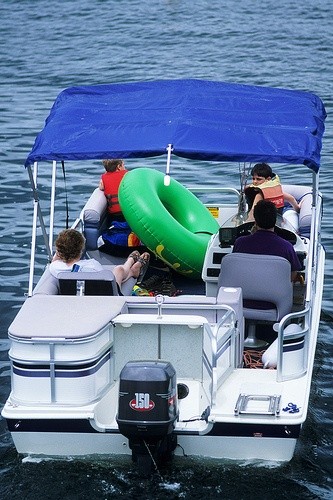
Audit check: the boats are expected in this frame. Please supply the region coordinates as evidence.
[1,78,328,481]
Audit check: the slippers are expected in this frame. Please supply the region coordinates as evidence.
[127,250,150,276]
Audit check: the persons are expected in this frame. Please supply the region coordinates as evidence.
[50,229,150,296]
[244,162,301,226]
[98,159,129,222]
[233,201,302,310]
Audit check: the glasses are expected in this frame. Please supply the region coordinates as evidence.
[252,178,263,181]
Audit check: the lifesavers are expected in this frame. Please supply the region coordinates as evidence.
[117,166,220,278]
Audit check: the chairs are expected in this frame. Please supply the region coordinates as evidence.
[217,253,292,349]
[56,270,135,296]
